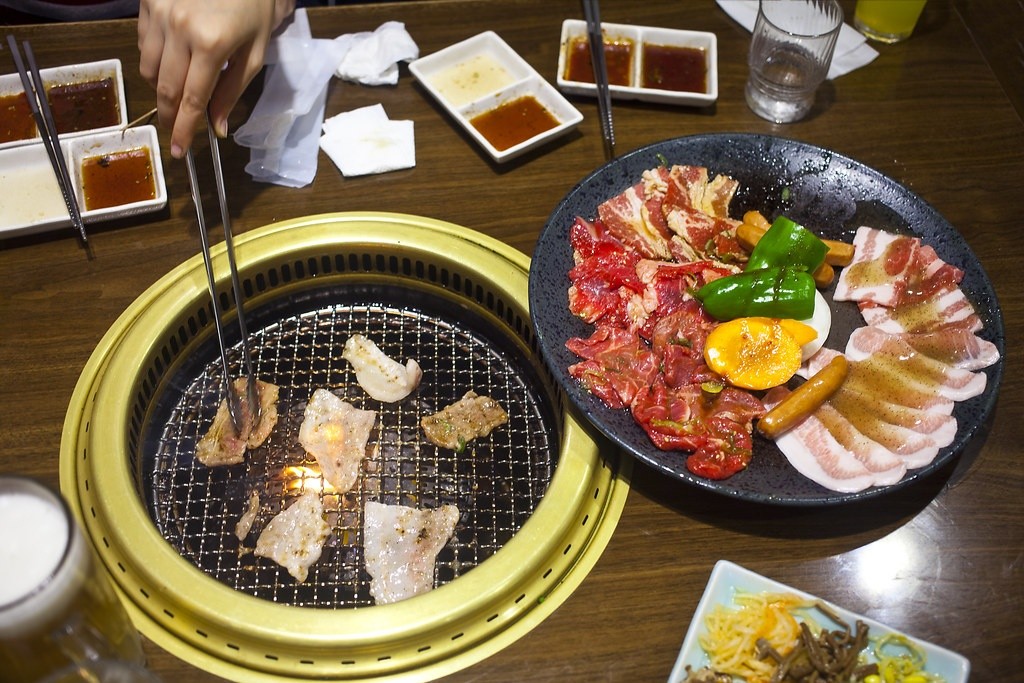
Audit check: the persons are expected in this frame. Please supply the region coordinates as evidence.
[134,0,299,160]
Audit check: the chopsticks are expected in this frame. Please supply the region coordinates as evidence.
[579,0,623,147]
[5,33,93,244]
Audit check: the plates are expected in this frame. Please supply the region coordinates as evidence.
[529,128,1007,510]
[555,17,721,106]
[0,119,166,246]
[407,26,586,162]
[658,557,972,682]
[0,56,134,151]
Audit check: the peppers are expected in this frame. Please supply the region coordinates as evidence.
[689,216,831,389]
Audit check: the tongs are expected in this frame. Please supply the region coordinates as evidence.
[166,65,266,432]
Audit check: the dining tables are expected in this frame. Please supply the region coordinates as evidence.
[1,2,1024,682]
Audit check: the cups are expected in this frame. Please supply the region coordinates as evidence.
[852,0,930,43]
[745,0,845,123]
[0,469,152,681]
